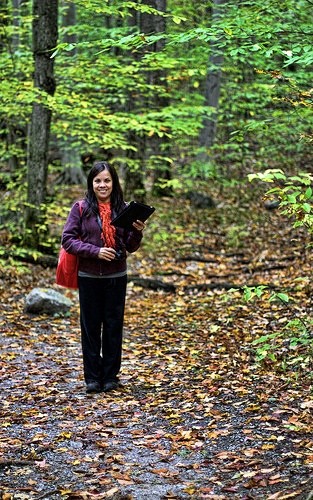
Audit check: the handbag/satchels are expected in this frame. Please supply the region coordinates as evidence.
[55,200,84,291]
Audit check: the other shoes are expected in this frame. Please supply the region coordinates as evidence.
[104,381,119,390]
[85,382,102,392]
